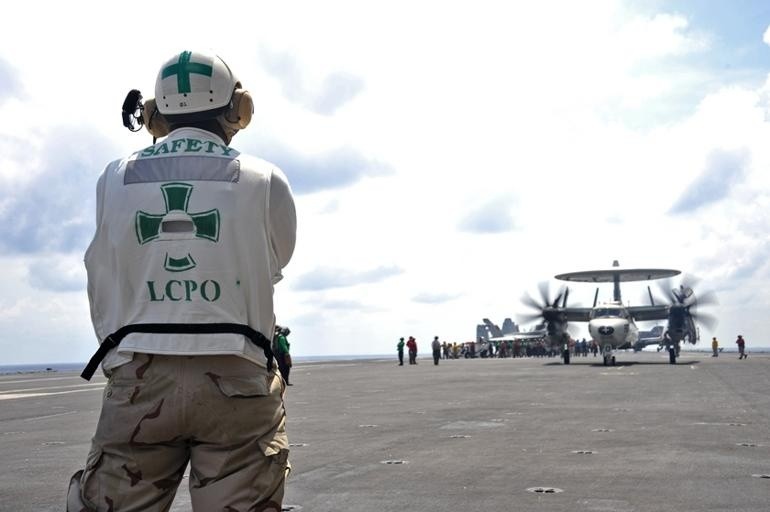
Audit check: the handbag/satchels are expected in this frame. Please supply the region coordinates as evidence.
[284,354,292,367]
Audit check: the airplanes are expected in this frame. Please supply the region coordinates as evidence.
[625,325,664,352]
[483,317,549,352]
[517,259,722,365]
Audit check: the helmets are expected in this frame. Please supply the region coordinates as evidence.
[153,50,243,117]
[274,324,291,336]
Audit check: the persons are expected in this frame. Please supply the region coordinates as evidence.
[736,336,746,359]
[67,51,296,512]
[397,336,602,366]
[712,338,718,357]
[277,328,293,386]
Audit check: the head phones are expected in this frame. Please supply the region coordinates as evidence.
[140,82,254,139]
